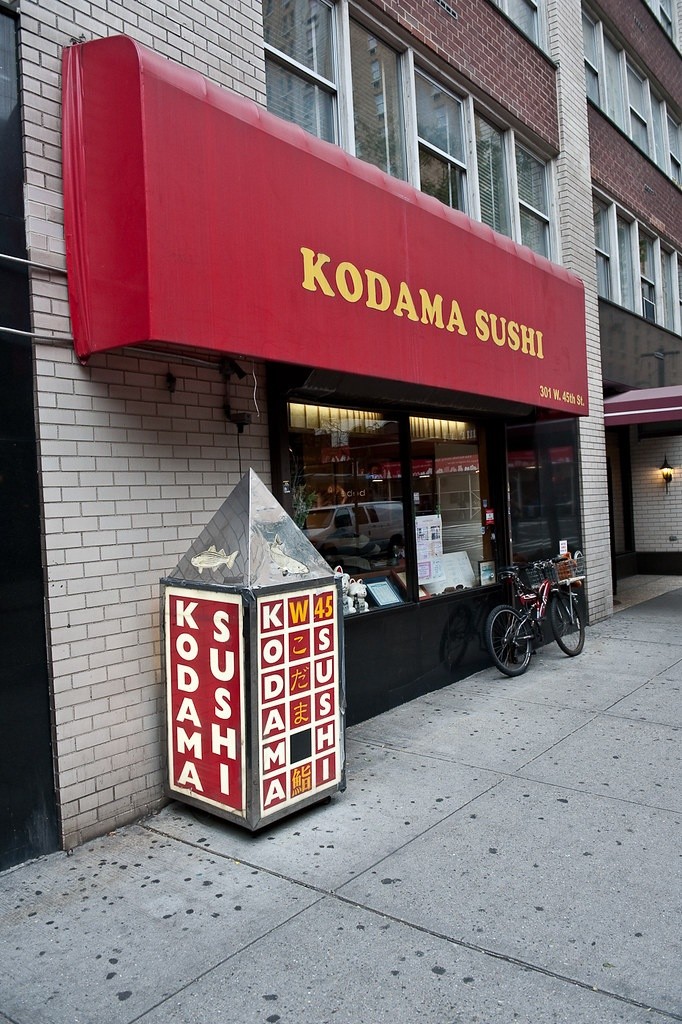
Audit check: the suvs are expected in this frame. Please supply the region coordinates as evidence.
[298,501,407,559]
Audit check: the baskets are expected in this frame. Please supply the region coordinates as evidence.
[526,556,587,588]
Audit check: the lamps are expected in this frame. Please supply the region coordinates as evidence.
[642,347,680,360]
[659,453,673,495]
[221,356,247,381]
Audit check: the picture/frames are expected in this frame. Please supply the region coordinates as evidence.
[363,576,404,607]
[392,567,432,600]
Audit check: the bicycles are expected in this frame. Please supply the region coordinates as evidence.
[440,596,494,671]
[487,562,585,677]
